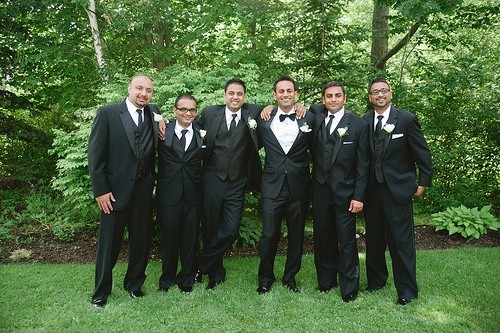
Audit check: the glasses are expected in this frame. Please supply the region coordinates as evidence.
[176,107,197,114]
[369,87,390,96]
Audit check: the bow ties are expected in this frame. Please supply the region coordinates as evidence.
[279,113,296,122]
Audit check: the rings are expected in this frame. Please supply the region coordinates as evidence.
[359,209,361,210]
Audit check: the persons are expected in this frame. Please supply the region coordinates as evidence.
[361,80,432,305]
[157,77,370,303]
[87,76,162,308]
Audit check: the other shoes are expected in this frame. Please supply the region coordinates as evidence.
[179,285,193,292]
[317,286,337,292]
[342,294,356,302]
[366,287,382,291]
[159,287,168,292]
[256,285,270,292]
[283,281,300,292]
[398,297,411,305]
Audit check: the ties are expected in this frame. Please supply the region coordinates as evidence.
[179,130,189,151]
[375,115,384,136]
[325,115,335,137]
[136,109,143,128]
[230,114,237,137]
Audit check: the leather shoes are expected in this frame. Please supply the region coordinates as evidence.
[91,299,107,307]
[195,268,203,283]
[131,291,145,298]
[205,280,224,290]
